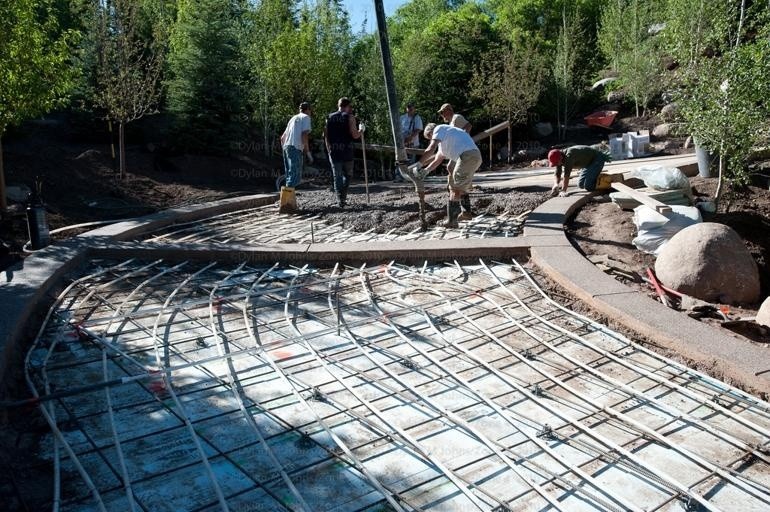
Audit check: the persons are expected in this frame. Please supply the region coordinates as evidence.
[393,102,424,183]
[408,123,483,226]
[324,97,366,207]
[281,101,314,189]
[438,103,472,198]
[548,145,606,197]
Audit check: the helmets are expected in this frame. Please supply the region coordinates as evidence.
[549,148,562,168]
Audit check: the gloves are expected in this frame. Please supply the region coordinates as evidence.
[550,184,569,197]
[357,122,366,132]
[406,161,430,179]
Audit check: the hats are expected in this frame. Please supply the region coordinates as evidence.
[406,100,453,114]
[298,97,352,109]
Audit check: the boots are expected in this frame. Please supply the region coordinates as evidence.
[437,193,472,229]
